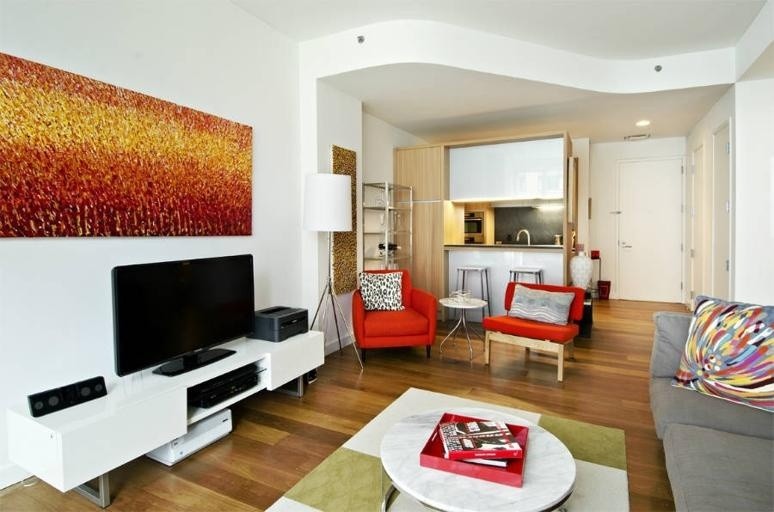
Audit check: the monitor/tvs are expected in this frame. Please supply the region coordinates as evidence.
[111,253,255,377]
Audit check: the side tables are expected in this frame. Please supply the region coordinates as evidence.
[439,297,486,362]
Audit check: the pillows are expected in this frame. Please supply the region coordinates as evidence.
[358,272,407,311]
[511,285,573,323]
[673,295,774,411]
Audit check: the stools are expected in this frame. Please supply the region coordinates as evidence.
[510,268,541,285]
[457,267,491,323]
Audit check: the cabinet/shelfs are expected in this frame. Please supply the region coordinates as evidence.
[360,178,415,270]
[4,330,324,507]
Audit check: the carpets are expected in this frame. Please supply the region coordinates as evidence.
[268,386,630,512]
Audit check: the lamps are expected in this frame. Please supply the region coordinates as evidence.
[303,174,366,369]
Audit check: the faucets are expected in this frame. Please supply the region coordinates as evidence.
[516,229,530,246]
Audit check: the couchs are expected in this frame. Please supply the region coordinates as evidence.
[484,283,584,381]
[352,269,437,361]
[647,311,774,511]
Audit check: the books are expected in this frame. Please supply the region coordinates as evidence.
[439,420,523,468]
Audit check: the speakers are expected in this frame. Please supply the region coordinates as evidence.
[27,376,108,418]
